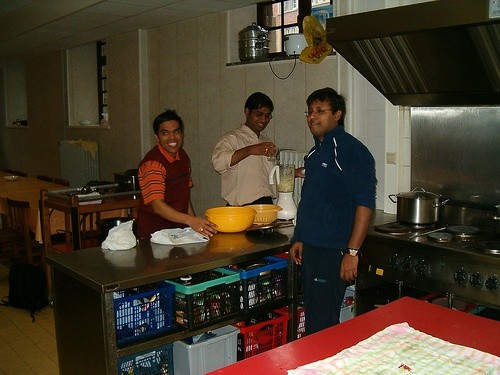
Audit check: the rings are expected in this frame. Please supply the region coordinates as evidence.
[200,227,203,231]
[266,149,268,151]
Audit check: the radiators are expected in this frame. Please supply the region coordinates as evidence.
[58,139,99,187]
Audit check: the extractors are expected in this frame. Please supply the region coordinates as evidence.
[326,0,500,107]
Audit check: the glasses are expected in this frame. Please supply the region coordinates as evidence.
[250,110,273,120]
[304,108,334,117]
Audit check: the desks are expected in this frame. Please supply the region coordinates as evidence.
[0,171,132,240]
[204,296,500,375]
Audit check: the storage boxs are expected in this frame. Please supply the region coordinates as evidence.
[110,253,371,375]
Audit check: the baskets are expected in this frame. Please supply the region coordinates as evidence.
[233,309,290,360]
[223,256,286,310]
[117,344,174,375]
[165,268,240,331]
[272,305,306,340]
[114,281,175,344]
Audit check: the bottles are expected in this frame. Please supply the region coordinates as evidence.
[100,113,109,125]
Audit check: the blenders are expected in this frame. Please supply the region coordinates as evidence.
[268,164,297,219]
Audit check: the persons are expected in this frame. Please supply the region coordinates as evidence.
[212,92,278,207]
[289,87,377,336]
[136,110,218,239]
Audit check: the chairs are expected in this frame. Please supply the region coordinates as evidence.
[0,169,116,309]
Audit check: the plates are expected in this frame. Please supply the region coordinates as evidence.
[4,176,18,181]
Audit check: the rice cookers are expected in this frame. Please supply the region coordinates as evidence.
[284,33,308,56]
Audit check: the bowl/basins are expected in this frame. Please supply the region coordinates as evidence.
[205,207,257,233]
[95,218,136,236]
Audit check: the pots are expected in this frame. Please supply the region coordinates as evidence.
[238,22,270,62]
[389,186,450,230]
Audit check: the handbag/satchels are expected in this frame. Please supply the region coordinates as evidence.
[6,257,48,311]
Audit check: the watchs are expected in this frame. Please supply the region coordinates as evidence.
[345,248,359,256]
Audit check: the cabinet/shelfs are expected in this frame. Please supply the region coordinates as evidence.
[40,187,141,305]
[47,220,355,375]
